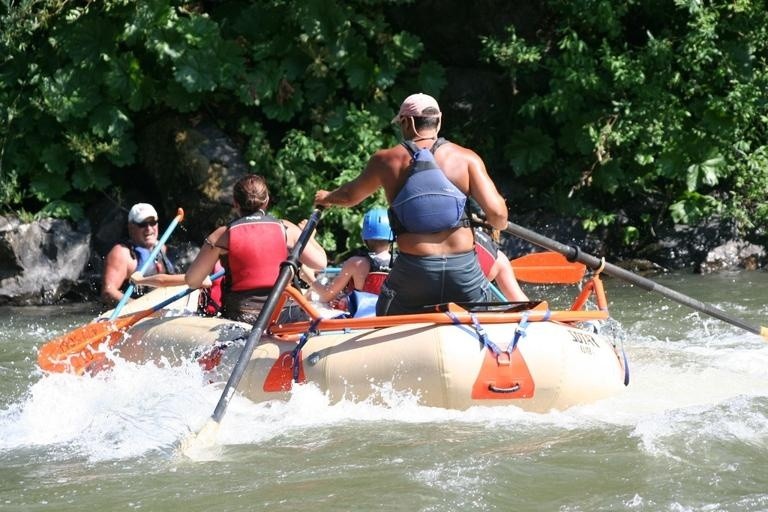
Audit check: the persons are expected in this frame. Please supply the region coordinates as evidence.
[130,256,227,316]
[185,174,327,328]
[449,222,530,315]
[101,203,178,308]
[318,93,510,313]
[297,205,397,316]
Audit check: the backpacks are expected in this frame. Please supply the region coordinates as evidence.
[388,138,468,232]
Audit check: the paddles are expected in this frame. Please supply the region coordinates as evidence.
[38,268,226,372]
[510,251,586,284]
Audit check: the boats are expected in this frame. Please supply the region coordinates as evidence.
[88,267,628,413]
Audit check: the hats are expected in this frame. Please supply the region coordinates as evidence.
[128,202,159,223]
[390,93,443,122]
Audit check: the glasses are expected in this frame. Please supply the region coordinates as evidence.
[130,222,157,227]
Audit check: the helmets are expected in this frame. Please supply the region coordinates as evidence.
[363,207,394,242]
